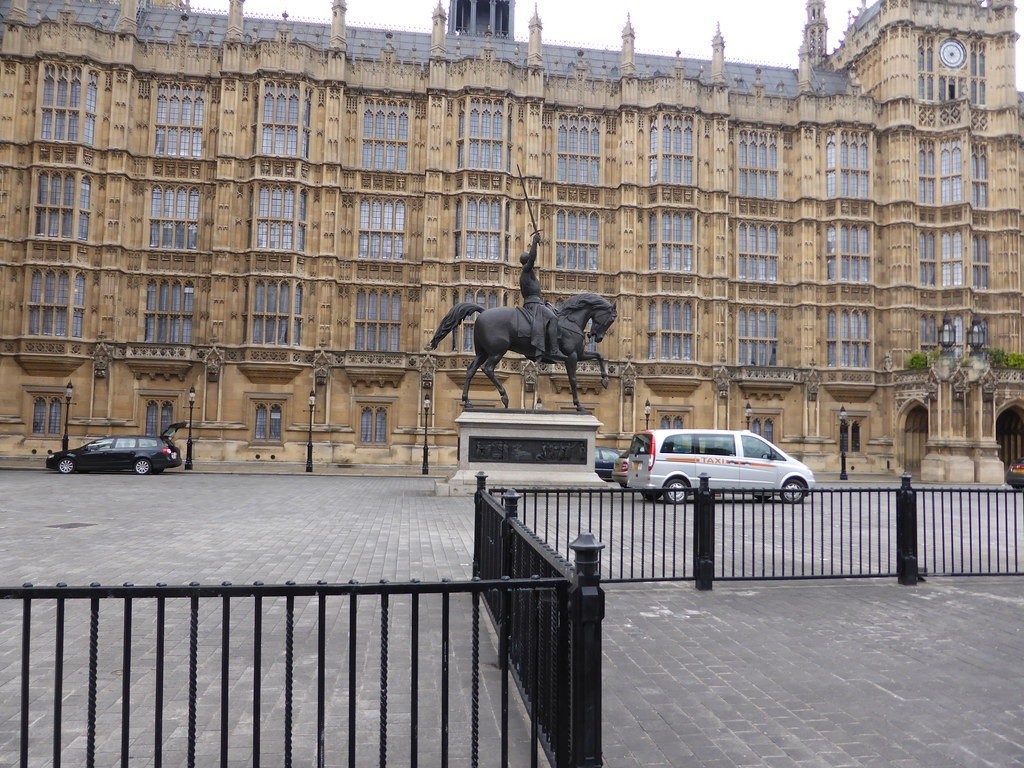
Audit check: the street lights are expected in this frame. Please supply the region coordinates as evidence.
[184,386,196,471]
[61,380,75,451]
[305,390,314,472]
[645,397,651,430]
[745,402,753,430]
[421,400,431,474]
[837,405,851,479]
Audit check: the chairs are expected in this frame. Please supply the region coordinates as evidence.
[704,440,715,454]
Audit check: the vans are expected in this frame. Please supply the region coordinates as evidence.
[627,428,816,503]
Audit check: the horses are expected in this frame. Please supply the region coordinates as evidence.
[430,293,617,411]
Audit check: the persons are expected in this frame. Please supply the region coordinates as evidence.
[518,234,568,364]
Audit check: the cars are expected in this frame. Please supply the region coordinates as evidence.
[1005,457,1024,488]
[46,422,189,476]
[612,448,628,488]
[594,447,624,480]
